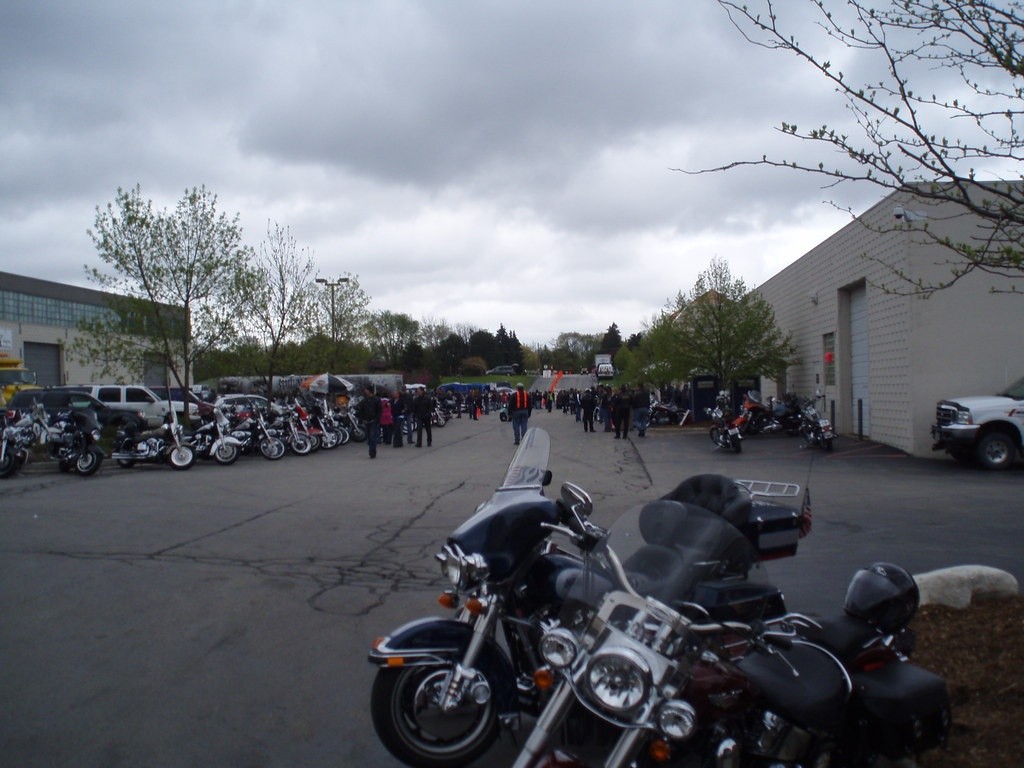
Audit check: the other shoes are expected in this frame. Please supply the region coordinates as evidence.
[585,428,588,432]
[590,429,596,432]
[514,442,519,445]
[638,429,646,436]
[614,436,621,439]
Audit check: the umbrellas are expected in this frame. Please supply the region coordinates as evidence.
[300,372,353,394]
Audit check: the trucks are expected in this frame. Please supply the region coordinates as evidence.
[0,351,41,407]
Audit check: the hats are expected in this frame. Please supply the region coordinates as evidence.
[517,383,523,387]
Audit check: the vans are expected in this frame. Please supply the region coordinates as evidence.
[147,385,216,417]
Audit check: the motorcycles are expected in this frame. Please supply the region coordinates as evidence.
[737,388,793,437]
[646,390,688,428]
[365,427,813,768]
[46,397,104,476]
[114,406,197,470]
[499,471,952,768]
[0,392,51,480]
[704,390,744,453]
[792,391,838,452]
[190,381,517,465]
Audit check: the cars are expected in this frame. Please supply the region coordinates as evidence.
[594,355,614,380]
[581,368,588,375]
[213,393,284,416]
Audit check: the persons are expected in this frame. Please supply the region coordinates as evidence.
[556,387,577,415]
[654,381,692,425]
[355,383,383,458]
[529,389,543,409]
[385,389,408,448]
[611,383,633,440]
[401,385,415,444]
[378,391,393,445]
[632,381,650,437]
[508,382,532,445]
[465,387,498,420]
[543,390,554,412]
[412,387,435,448]
[574,389,596,432]
[496,390,510,409]
[591,384,614,432]
[447,388,464,419]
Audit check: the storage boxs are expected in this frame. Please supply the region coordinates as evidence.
[858,662,952,763]
[749,499,799,559]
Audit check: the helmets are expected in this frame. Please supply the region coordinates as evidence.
[843,560,920,634]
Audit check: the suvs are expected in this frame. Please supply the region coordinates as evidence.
[6,388,149,432]
[929,375,1024,473]
[486,365,514,376]
[64,383,199,430]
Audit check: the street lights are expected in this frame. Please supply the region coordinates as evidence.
[316,277,350,374]
